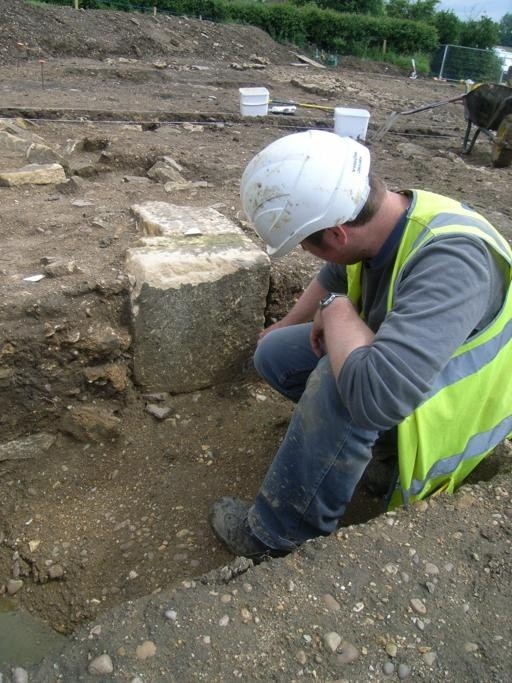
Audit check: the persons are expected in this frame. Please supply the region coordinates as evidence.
[208,128,506,562]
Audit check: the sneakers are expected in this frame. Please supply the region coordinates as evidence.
[209,496,276,560]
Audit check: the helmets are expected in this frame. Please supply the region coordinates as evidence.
[238,129,371,258]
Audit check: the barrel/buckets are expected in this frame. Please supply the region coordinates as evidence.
[238,87,270,115]
[332,106,370,140]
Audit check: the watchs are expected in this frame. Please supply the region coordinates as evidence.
[318,292,348,308]
